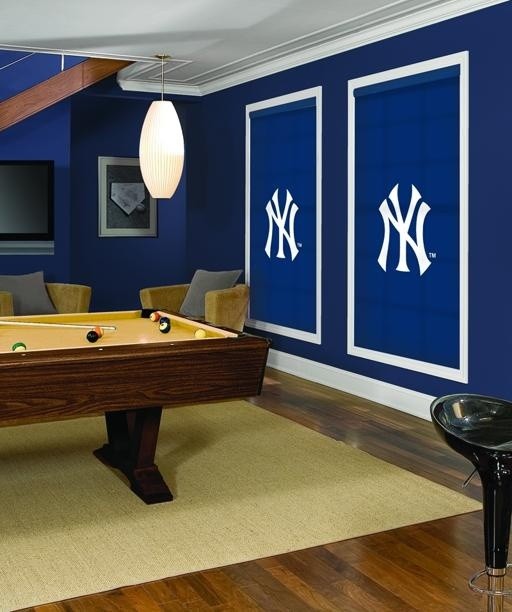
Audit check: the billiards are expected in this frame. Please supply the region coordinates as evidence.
[195,330,205,337]
[86,327,103,343]
[150,312,170,333]
[13,343,26,352]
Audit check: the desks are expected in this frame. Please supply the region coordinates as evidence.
[0,306,273,506]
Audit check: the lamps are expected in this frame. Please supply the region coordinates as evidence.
[136,50,187,203]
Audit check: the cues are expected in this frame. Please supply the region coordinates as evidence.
[0,321,116,330]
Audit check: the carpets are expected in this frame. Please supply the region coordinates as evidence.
[0,399,482,610]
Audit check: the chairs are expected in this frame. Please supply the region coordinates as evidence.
[0,280,93,316]
[137,280,250,333]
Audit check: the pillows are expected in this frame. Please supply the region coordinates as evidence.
[177,263,244,322]
[1,269,59,316]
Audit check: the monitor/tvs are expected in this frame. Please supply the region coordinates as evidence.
[1,161,54,248]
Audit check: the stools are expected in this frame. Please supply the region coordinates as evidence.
[430,392,512,612]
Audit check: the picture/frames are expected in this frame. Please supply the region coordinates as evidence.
[93,153,160,240]
